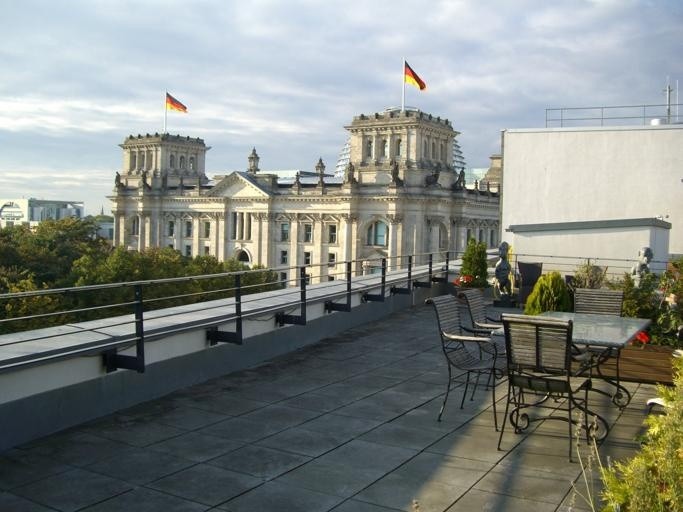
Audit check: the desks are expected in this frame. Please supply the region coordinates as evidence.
[505,311,652,440]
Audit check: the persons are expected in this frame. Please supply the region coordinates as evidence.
[494,242,512,297]
[629,247,652,288]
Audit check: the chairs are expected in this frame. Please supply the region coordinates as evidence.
[460,289,527,402]
[424,295,506,431]
[634,349,683,440]
[573,288,624,401]
[497,314,591,462]
[518,262,542,310]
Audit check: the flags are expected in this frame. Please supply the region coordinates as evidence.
[404,61,426,94]
[165,93,187,115]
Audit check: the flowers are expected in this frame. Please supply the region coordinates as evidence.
[453,274,474,288]
